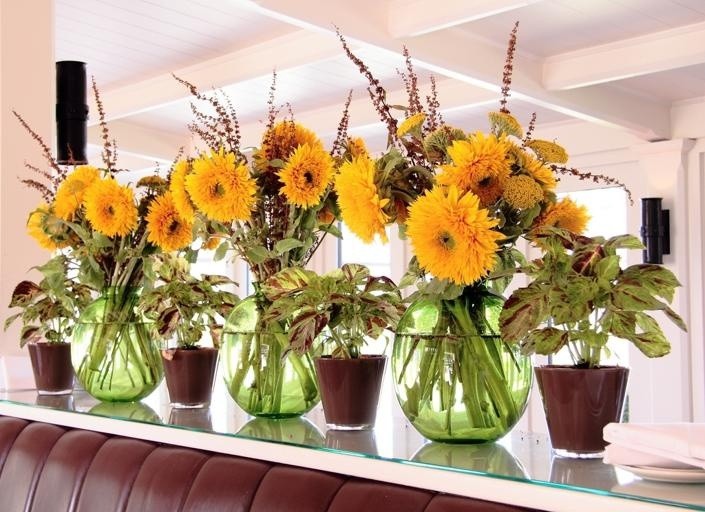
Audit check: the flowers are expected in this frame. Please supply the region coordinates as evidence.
[317,20,636,431]
[16,84,188,389]
[144,68,375,413]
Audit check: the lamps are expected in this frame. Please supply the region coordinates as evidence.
[638,196,673,268]
[53,60,93,167]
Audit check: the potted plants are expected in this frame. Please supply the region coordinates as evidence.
[264,259,407,429]
[501,221,687,459]
[4,275,76,393]
[140,272,241,408]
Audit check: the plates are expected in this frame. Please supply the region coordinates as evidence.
[615,463,705,485]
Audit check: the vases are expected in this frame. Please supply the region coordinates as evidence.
[220,291,324,419]
[389,288,535,446]
[63,285,168,403]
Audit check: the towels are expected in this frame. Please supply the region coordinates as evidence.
[598,418,705,470]
[602,445,694,470]
